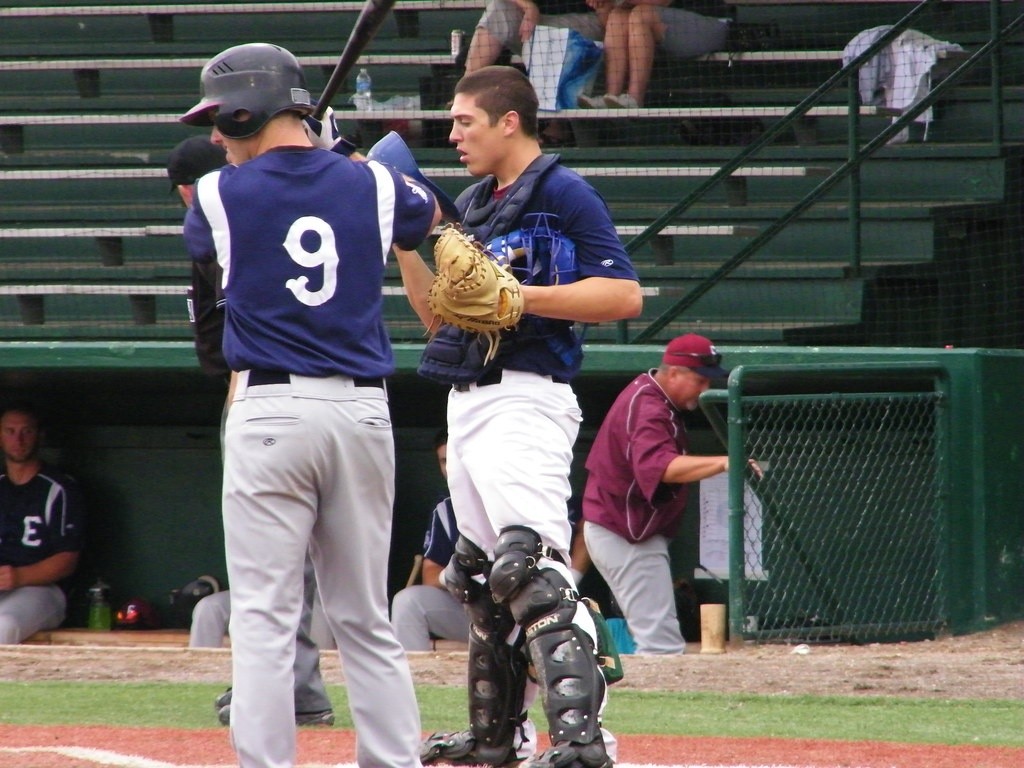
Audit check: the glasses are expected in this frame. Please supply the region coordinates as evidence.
[671,347,723,366]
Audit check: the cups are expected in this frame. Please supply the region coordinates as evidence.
[700,604,727,654]
[451,29,466,55]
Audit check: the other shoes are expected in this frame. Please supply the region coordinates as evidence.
[604,94,639,110]
[217,687,236,705]
[219,707,335,726]
[576,92,606,107]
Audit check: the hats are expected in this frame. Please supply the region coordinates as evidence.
[169,136,231,190]
[665,332,727,376]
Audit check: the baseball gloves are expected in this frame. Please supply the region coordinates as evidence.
[427,227,524,334]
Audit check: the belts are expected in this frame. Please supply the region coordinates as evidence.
[248,371,384,389]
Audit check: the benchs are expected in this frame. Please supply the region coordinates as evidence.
[0,0,1024,341]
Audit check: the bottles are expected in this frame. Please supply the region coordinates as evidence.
[355,69,372,110]
[87,577,112,631]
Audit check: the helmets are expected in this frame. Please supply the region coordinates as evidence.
[478,229,583,350]
[179,43,315,141]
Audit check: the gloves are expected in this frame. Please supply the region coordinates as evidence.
[302,99,357,158]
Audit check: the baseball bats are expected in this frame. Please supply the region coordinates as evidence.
[312,0,396,121]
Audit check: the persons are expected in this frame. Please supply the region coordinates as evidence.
[391,67,643,768]
[182,41,442,768]
[0,405,86,647]
[168,133,338,728]
[390,428,595,652]
[447,0,620,147]
[581,334,764,657]
[577,0,735,108]
